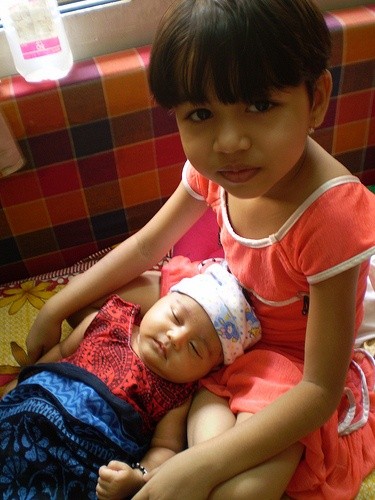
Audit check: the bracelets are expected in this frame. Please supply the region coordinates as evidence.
[130,462,148,477]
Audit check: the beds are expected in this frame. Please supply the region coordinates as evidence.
[0,189,375,500]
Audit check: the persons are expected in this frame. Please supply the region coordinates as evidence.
[25,0,373,499]
[0,264,261,500]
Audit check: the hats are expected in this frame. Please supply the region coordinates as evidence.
[168,263,261,366]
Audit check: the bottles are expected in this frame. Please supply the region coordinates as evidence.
[0,0,74,83]
